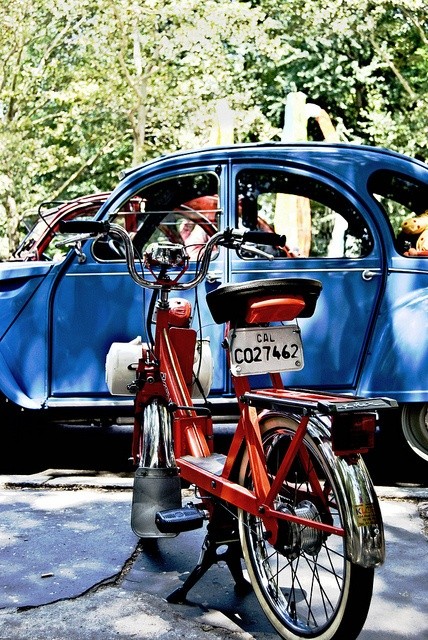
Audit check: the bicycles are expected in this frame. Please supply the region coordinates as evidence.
[57,223,399,640]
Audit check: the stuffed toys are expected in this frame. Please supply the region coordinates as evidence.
[401,210,427,256]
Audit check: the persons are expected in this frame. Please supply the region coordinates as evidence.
[178,218,217,261]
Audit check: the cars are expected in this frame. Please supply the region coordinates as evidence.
[5,191,295,261]
[1,142,427,484]
